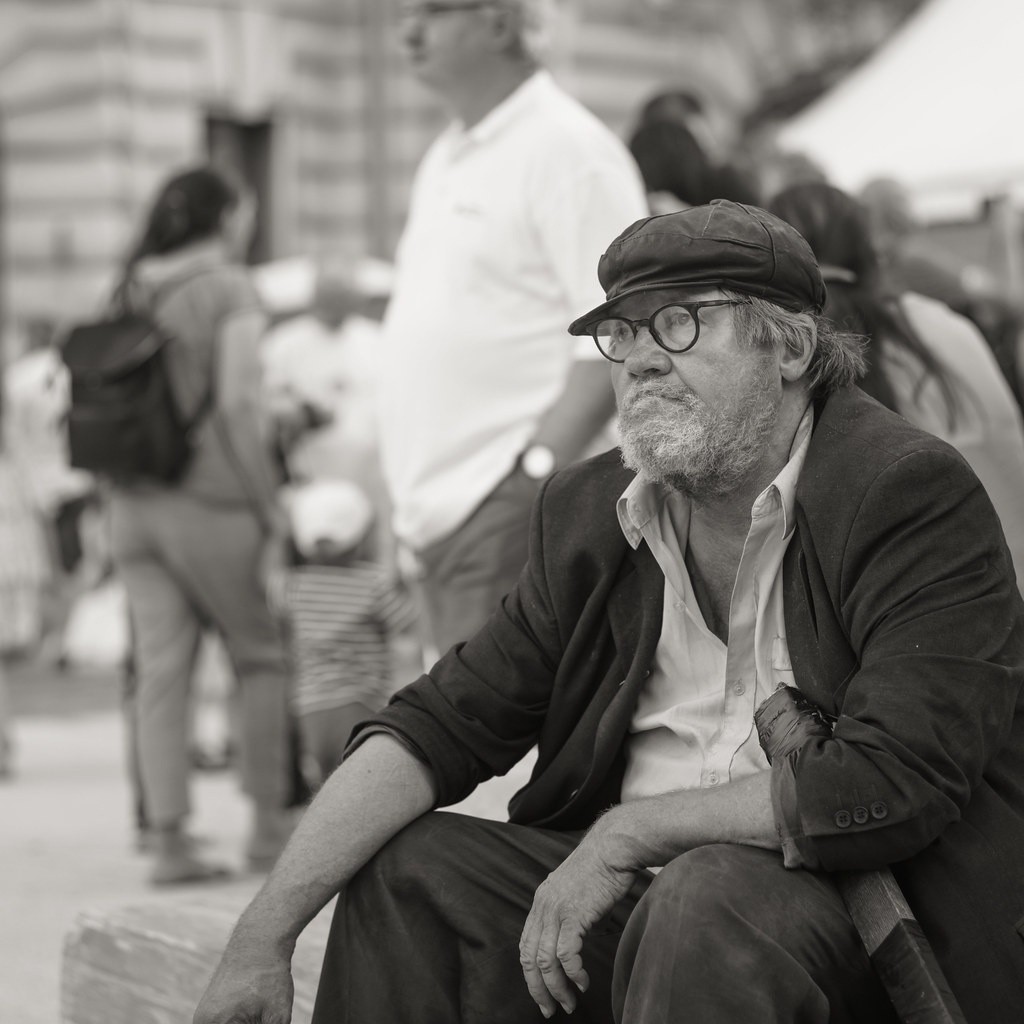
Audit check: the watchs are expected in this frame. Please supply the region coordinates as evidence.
[521,446,555,480]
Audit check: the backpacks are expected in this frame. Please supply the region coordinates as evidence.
[61,261,216,492]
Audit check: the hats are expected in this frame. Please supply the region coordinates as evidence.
[568,199,827,336]
[291,478,373,558]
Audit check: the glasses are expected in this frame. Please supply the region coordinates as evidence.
[585,299,752,363]
[400,0,484,22]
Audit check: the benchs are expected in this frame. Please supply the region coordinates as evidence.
[60,896,332,1024]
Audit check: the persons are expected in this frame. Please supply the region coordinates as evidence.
[626,93,970,323]
[109,169,309,885]
[2,318,93,577]
[770,186,1024,599]
[193,199,1024,1023]
[377,0,650,662]
[280,479,418,792]
